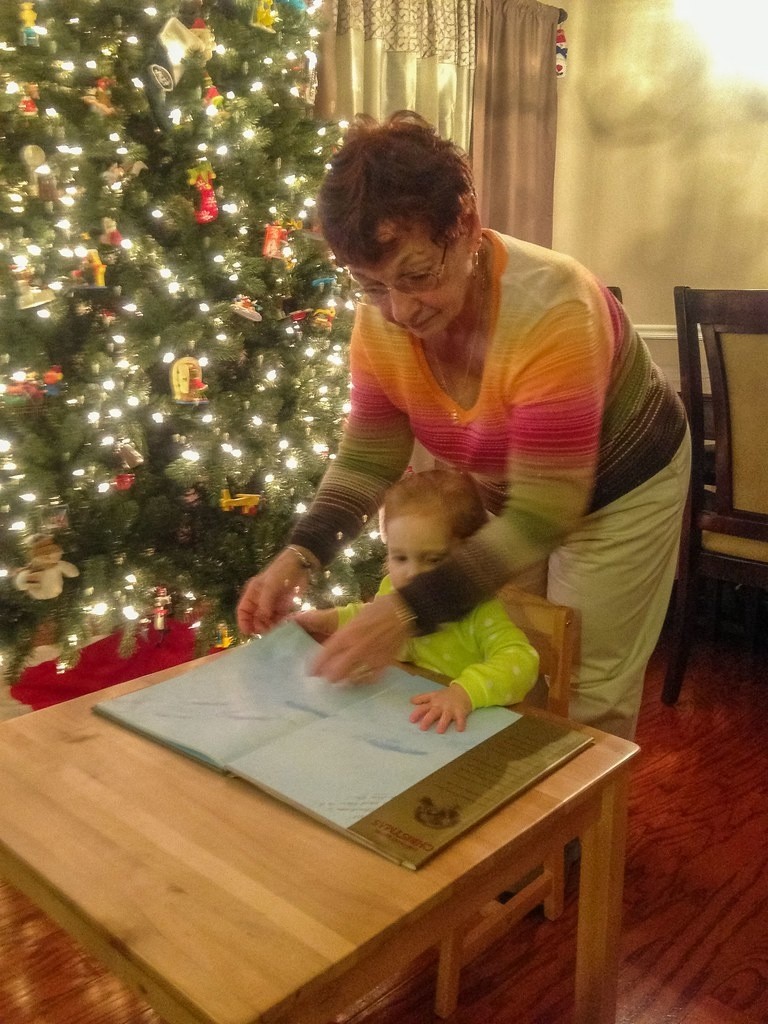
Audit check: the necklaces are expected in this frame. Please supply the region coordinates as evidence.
[432,249,487,425]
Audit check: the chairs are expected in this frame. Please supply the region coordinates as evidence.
[433,600,579,1020]
[656,284,768,707]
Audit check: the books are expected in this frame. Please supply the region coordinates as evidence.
[94,618,594,872]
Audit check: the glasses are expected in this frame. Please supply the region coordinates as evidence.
[347,241,449,305]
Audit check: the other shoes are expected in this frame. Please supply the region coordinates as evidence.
[499,887,543,914]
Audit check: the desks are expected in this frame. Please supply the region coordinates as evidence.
[0,628,642,1024]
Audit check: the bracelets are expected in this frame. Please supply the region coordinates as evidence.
[286,546,319,585]
[388,592,418,633]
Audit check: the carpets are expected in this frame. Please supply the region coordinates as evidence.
[9,598,233,715]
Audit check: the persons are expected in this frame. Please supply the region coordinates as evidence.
[233,108,692,909]
[295,469,541,735]
